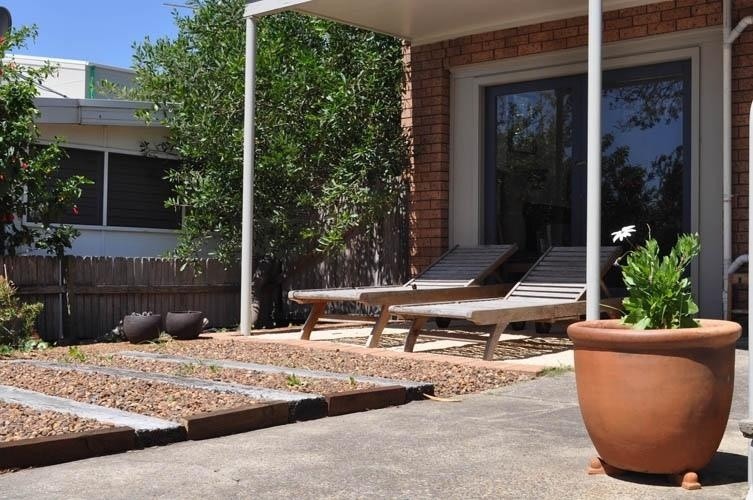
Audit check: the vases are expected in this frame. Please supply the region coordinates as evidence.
[568,317,744,488]
[123,309,203,345]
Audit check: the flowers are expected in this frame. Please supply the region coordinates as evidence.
[611,221,705,329]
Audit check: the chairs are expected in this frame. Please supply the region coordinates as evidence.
[388,245,625,360]
[286,242,520,349]
